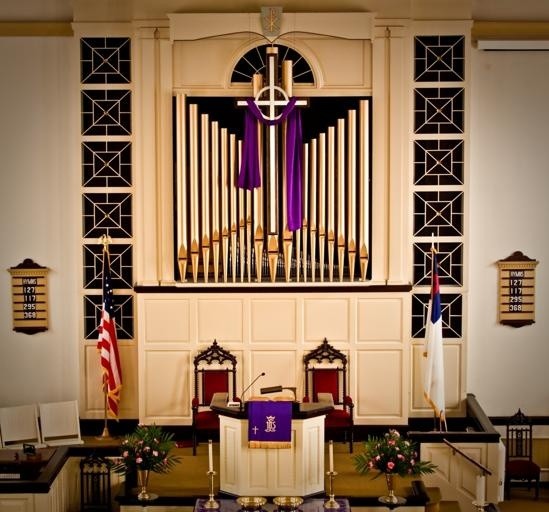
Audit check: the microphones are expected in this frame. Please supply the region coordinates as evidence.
[240,372,265,408]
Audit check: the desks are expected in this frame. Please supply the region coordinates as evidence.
[194,498,348,512]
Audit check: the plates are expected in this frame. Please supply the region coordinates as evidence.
[236,497,267,510]
[273,497,304,509]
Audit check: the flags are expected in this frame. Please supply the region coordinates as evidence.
[420,248,446,432]
[97,249,123,425]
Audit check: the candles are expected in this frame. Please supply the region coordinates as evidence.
[208,439,213,471]
[329,439,334,473]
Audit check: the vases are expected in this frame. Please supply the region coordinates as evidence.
[384,473,397,504]
[137,469,151,501]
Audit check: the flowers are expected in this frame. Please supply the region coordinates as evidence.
[351,429,439,480]
[109,420,184,476]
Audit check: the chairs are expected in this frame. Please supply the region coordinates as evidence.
[80,454,113,512]
[39,400,85,447]
[0,404,46,449]
[303,338,354,453]
[506,408,540,501]
[192,339,242,456]
